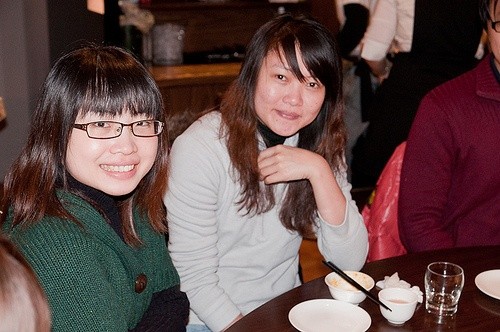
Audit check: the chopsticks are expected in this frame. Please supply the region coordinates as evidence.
[320,259,392,312]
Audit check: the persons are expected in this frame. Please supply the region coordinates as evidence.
[160,14,370,332]
[0,42,190,332]
[335,0,488,216]
[397,0,500,253]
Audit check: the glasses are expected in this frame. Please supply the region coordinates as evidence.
[71,118,166,139]
[485,5,500,33]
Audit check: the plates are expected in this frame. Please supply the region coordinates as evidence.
[474,269,500,300]
[288,299,372,332]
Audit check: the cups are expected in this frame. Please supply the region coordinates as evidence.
[152,23,185,64]
[424,261,465,317]
[377,287,418,324]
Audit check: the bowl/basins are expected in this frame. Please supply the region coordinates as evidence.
[324,271,376,306]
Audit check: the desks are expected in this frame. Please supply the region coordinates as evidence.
[225,245,500,332]
